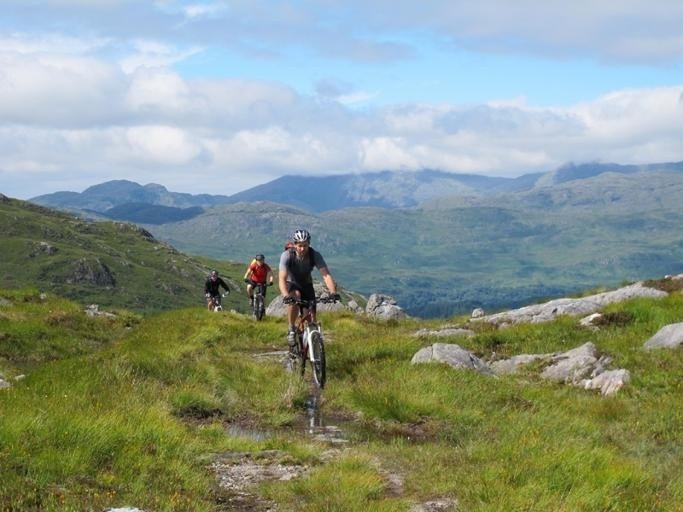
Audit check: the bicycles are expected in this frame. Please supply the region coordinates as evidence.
[244,278,273,321]
[205,293,229,313]
[283,295,342,388]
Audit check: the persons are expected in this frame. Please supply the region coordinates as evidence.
[275,229,338,361]
[243,253,273,316]
[203,270,230,310]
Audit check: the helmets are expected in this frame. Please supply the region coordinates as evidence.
[293,230,310,243]
[256,255,265,260]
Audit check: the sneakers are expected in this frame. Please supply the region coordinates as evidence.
[288,331,296,346]
[249,298,253,306]
